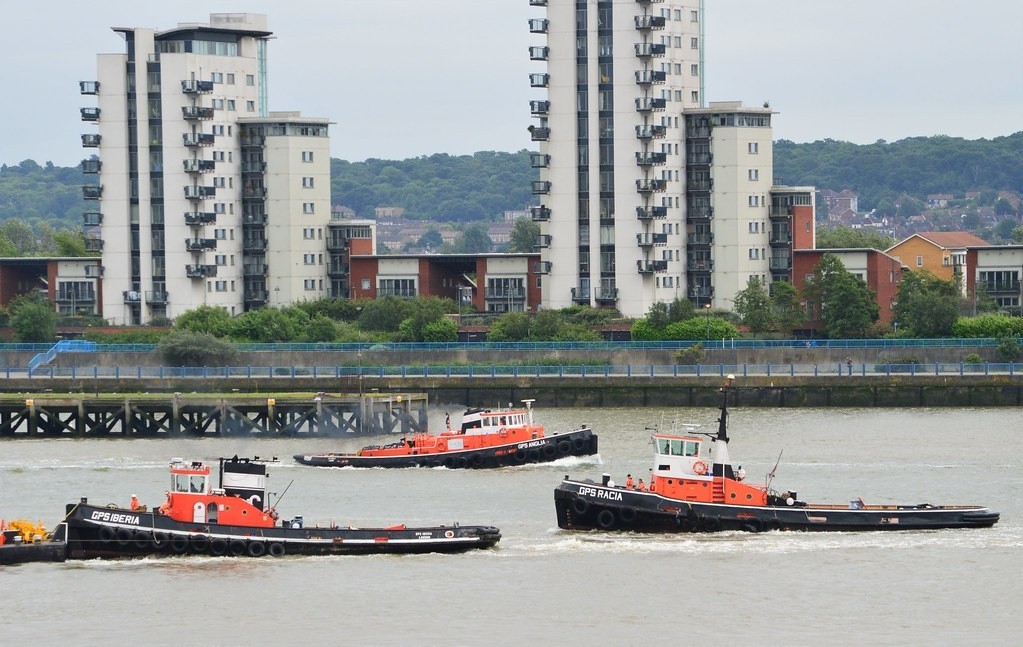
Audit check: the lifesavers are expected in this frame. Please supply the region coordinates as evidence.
[158,505,172,517]
[436,442,446,452]
[498,427,508,438]
[692,460,706,475]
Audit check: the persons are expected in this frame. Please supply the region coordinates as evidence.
[625,474,635,491]
[846,357,853,376]
[637,478,645,491]
[130,494,139,511]
[158,490,173,516]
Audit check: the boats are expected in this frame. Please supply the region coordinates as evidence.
[0,521,66,565]
[292,396,598,469]
[62,455,502,559]
[551,389,1001,530]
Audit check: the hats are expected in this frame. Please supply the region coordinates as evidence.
[131,494,136,497]
